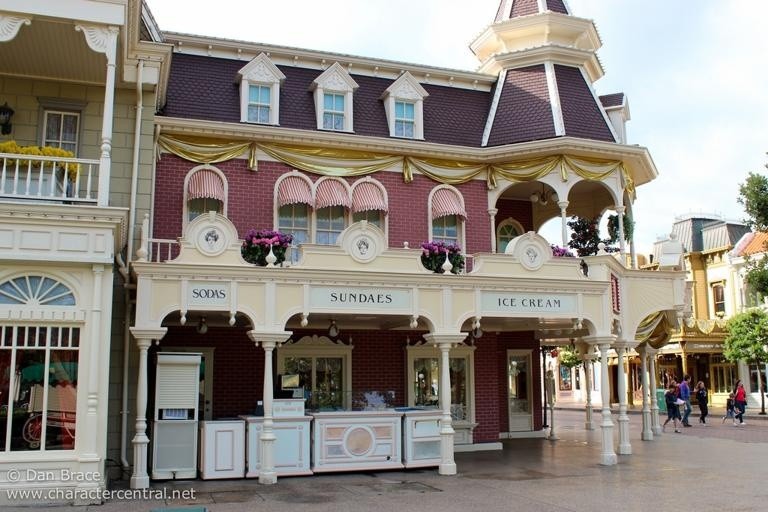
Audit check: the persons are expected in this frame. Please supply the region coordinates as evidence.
[662,374,708,433]
[721,379,748,426]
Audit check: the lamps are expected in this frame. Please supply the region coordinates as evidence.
[0,101,15,135]
[328,319,339,337]
[196,315,208,334]
[472,328,483,338]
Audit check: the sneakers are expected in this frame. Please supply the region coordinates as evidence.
[733,418,746,427]
[699,416,702,423]
[672,429,681,433]
[661,424,665,433]
[703,423,706,427]
[682,422,692,427]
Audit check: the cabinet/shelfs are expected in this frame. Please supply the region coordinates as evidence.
[198,398,442,481]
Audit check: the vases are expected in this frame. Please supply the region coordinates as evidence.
[0,165,65,204]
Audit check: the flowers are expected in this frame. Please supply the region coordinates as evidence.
[419,240,465,274]
[241,229,296,266]
[551,215,636,276]
[0,140,83,183]
